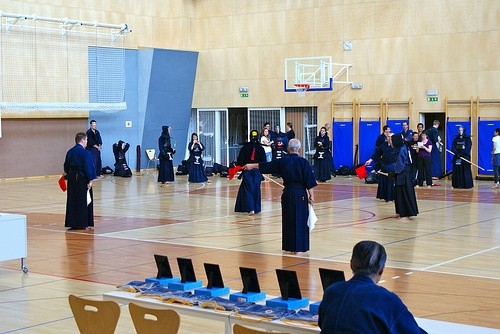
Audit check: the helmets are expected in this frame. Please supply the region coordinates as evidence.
[250,129,259,145]
[118,141,125,150]
[162,126,172,138]
[391,134,404,153]
[459,126,465,136]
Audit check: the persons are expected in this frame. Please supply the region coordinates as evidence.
[318,241,428,334]
[187,133,212,184]
[234,129,267,215]
[451,125,474,189]
[260,122,296,178]
[364,119,443,220]
[313,127,330,182]
[113,140,132,177]
[242,138,319,255]
[64,132,97,229]
[158,126,176,183]
[85,120,105,179]
[318,123,335,176]
[491,128,500,188]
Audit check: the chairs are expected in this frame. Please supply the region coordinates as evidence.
[68,294,120,334]
[128,302,180,334]
[145,147,160,171]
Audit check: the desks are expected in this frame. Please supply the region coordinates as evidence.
[102,279,500,334]
